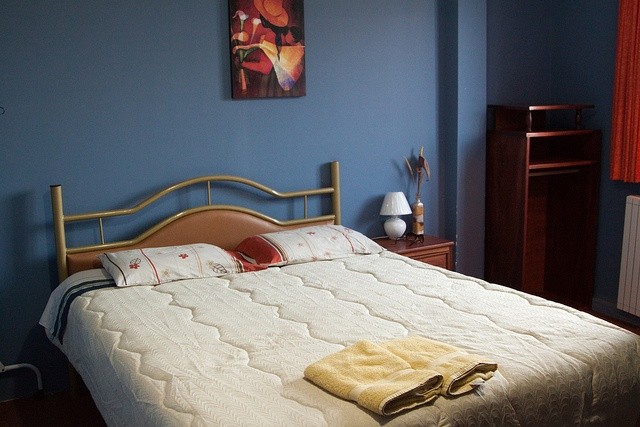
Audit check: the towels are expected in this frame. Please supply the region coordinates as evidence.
[379,334,500,399]
[302,339,444,417]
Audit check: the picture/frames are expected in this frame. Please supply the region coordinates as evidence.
[227,0,307,100]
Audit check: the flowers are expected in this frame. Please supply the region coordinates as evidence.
[403,146,431,199]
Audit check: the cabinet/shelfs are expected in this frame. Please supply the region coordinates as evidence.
[486,104,604,313]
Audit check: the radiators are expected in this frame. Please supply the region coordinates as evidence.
[616,194,640,319]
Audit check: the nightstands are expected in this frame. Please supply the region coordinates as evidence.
[373,233,455,271]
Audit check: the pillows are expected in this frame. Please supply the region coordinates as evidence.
[234,224,385,266]
[95,243,269,287]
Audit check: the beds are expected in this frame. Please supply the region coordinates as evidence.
[37,161,640,427]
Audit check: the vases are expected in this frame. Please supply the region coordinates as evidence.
[410,198,425,235]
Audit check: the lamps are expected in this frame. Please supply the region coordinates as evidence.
[379,192,413,240]
